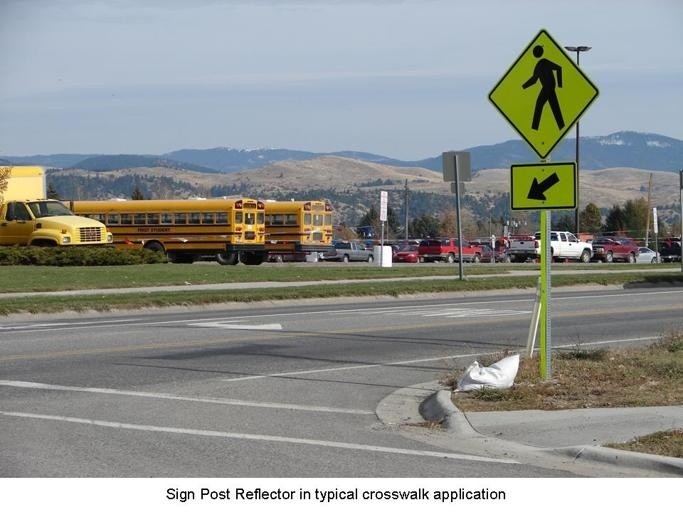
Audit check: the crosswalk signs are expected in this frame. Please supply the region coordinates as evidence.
[489,31,598,157]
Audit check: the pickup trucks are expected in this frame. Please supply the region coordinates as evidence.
[504,231,594,262]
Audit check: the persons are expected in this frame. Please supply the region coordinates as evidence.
[124,236,134,244]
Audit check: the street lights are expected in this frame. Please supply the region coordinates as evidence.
[564,46,592,233]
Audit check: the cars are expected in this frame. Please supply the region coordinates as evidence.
[319,237,509,264]
[592,236,661,263]
